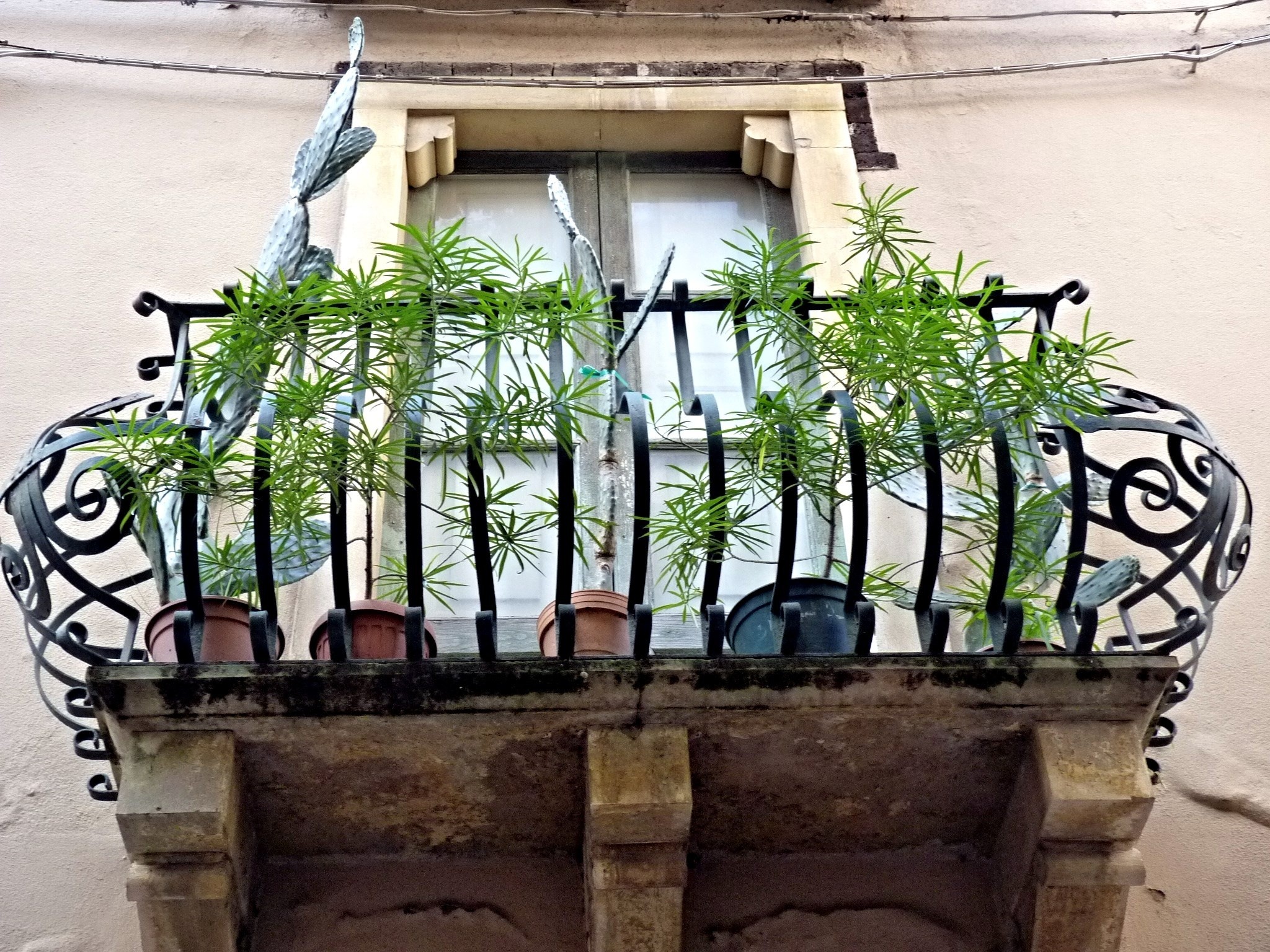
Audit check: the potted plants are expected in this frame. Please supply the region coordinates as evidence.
[75,15,377,662]
[624,183,1136,664]
[870,370,1143,655]
[78,187,633,666]
[512,175,687,660]
